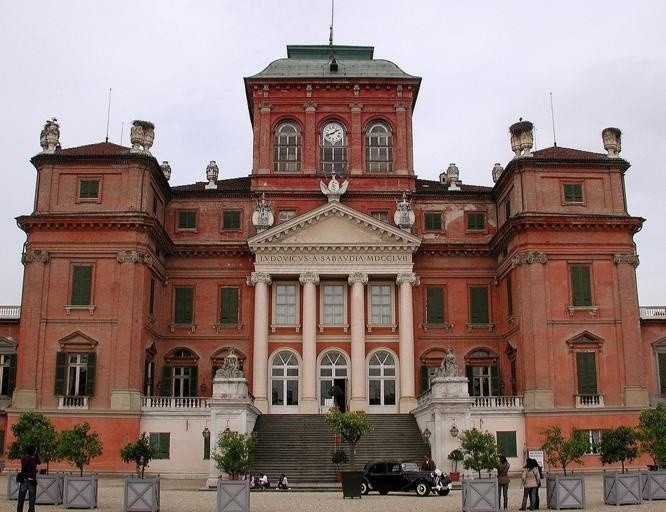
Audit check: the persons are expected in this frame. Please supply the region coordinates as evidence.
[531,459,544,510]
[495,455,511,510]
[242,473,255,487]
[518,459,541,509]
[275,474,291,490]
[257,471,270,489]
[215,348,245,378]
[421,454,437,494]
[441,346,457,369]
[15,445,41,511]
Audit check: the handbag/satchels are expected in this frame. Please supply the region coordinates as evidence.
[15,471,25,483]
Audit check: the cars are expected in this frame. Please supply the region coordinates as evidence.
[360,460,452,496]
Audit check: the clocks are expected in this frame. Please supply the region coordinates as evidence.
[324,123,344,145]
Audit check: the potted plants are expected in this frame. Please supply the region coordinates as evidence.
[8,410,47,499]
[332,451,349,482]
[539,424,594,511]
[601,425,644,506]
[324,406,374,500]
[0,460,6,474]
[324,408,344,445]
[56,422,103,509]
[448,449,464,480]
[119,431,160,511]
[637,398,666,501]
[458,428,503,511]
[32,425,63,505]
[209,425,258,512]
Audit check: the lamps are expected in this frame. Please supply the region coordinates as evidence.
[200,379,206,391]
[449,419,459,436]
[423,420,435,444]
